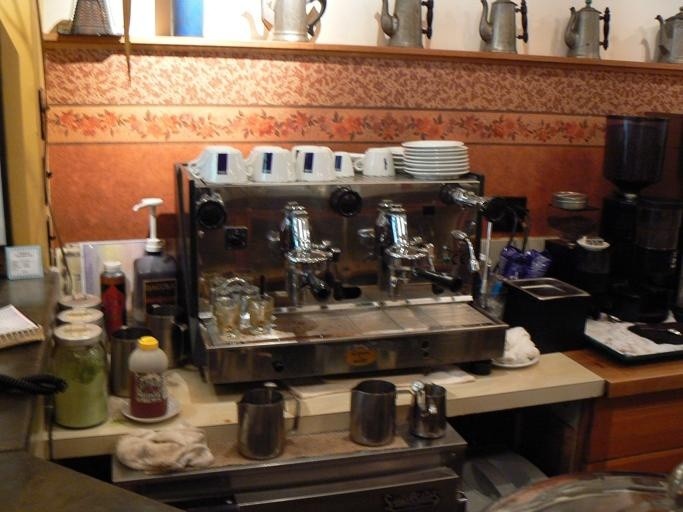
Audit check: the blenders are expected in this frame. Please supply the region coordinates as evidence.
[598,114,683,323]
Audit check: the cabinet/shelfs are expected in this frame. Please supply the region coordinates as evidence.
[513,349,683,477]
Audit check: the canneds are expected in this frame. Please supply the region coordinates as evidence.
[57,291,102,309]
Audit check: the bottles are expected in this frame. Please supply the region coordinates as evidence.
[127,335,166,418]
[100,260,128,335]
[58,293,102,311]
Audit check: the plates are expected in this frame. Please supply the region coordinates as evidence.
[491,351,540,369]
[119,397,180,423]
[390,140,470,180]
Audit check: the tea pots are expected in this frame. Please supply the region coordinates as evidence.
[478,0,527,54]
[408,384,446,438]
[654,6,683,64]
[262,0,326,42]
[349,379,413,446]
[380,0,433,49]
[563,0,610,58]
[235,387,300,460]
[145,303,188,368]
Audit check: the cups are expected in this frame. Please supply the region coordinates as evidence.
[354,148,395,177]
[185,144,354,185]
[212,299,241,343]
[110,328,152,397]
[247,295,273,335]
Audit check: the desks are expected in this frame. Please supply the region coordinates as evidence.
[27,346,607,462]
